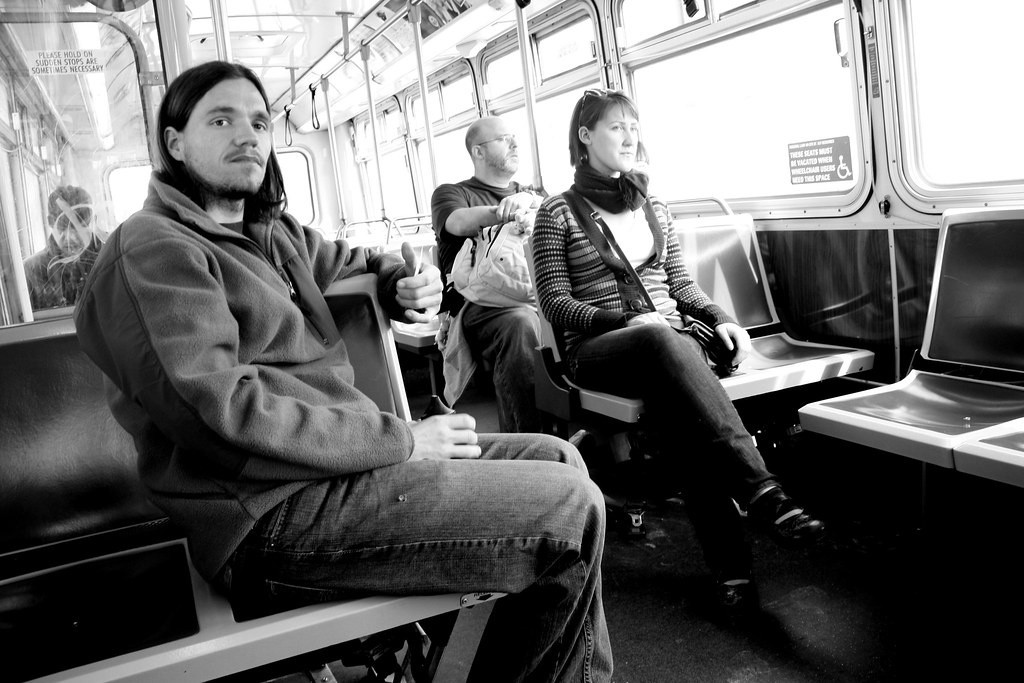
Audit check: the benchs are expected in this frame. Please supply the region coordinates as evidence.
[336,195,876,565]
[0,272,508,683]
[800,201,1024,492]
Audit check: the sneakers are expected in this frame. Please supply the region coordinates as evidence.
[715,566,757,611]
[746,485,825,548]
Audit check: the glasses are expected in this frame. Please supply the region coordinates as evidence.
[578,89,619,127]
[478,133,518,145]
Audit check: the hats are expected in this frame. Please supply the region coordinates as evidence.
[48,185,93,227]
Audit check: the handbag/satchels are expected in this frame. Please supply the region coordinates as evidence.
[682,313,740,376]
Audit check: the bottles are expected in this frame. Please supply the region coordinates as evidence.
[420,394,456,419]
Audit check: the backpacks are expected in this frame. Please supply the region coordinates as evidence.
[449,217,537,311]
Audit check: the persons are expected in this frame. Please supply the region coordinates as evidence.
[531,89,826,619]
[429,116,556,434]
[73,60,613,683]
[22,185,108,312]
